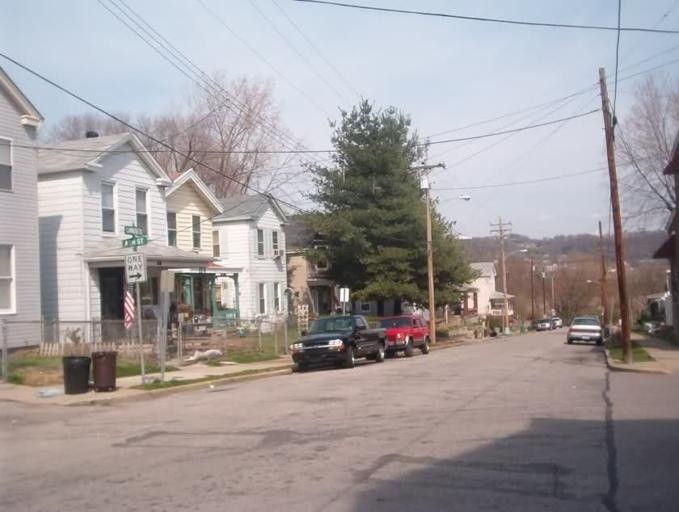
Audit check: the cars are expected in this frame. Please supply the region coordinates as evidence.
[536,316,563,332]
[566,317,602,346]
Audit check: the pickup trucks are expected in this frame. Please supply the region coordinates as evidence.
[289,314,430,368]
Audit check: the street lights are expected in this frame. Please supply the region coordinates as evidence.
[501,249,529,335]
[587,279,599,286]
[426,196,471,346]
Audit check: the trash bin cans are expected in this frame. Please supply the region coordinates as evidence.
[62,351,117,394]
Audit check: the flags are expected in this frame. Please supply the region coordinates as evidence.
[122,283,136,331]
